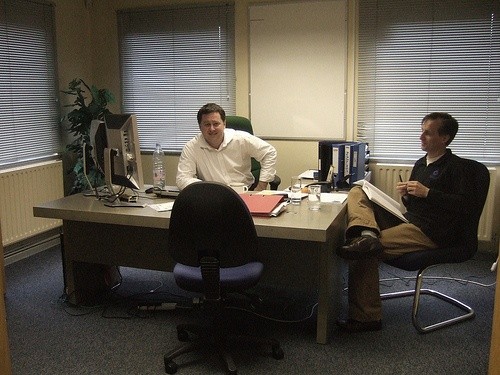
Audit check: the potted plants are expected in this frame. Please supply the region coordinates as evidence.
[56,77,117,196]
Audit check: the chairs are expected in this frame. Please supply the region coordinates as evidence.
[378,159,491,334]
[226,116,281,191]
[164,181,285,375]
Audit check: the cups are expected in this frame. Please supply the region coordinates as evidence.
[308,185,321,210]
[291,176,303,205]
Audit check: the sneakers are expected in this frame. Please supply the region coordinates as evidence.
[338,234,385,257]
[335,318,383,331]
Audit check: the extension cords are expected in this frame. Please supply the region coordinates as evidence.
[138,303,177,311]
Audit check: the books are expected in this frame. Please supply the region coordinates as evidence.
[353,179,409,223]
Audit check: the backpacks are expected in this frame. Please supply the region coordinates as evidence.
[72,259,123,299]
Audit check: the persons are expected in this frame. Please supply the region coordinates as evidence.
[336,112,474,330]
[175,103,277,191]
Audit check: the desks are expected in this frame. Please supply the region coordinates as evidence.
[32,169,372,345]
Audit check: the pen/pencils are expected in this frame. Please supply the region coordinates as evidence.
[398,174,402,182]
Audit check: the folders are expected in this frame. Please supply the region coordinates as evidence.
[237,194,285,216]
[316,140,370,190]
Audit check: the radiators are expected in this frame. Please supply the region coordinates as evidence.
[374,163,497,241]
[0,160,64,247]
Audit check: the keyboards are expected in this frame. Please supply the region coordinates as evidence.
[154,190,180,198]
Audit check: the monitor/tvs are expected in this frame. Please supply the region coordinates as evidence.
[82,114,147,208]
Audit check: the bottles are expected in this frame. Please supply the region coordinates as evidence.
[152,143,167,190]
